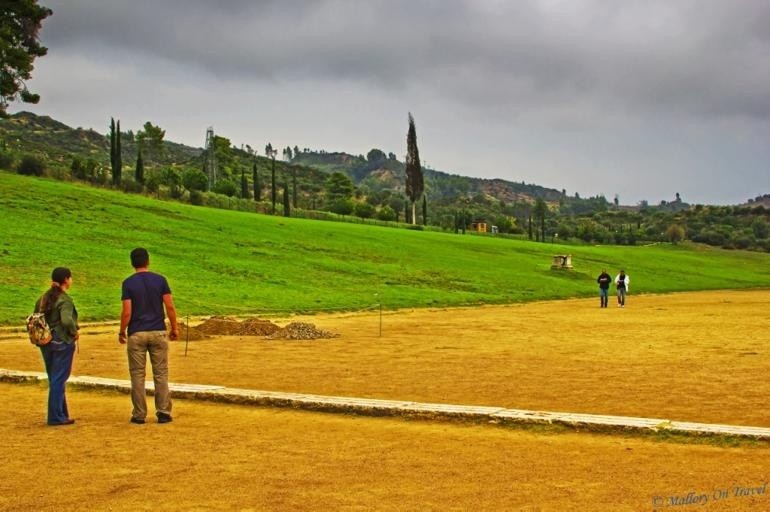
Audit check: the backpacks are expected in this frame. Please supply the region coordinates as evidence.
[26,294,60,346]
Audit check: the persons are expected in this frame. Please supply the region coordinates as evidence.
[597,267,612,309]
[119,246,180,424]
[614,271,630,308]
[34,267,80,426]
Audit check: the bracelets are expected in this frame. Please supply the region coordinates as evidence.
[119,331,124,337]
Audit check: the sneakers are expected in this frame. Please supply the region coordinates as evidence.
[601,303,607,307]
[48,417,74,424]
[131,416,145,423]
[617,303,624,307]
[158,412,172,423]
